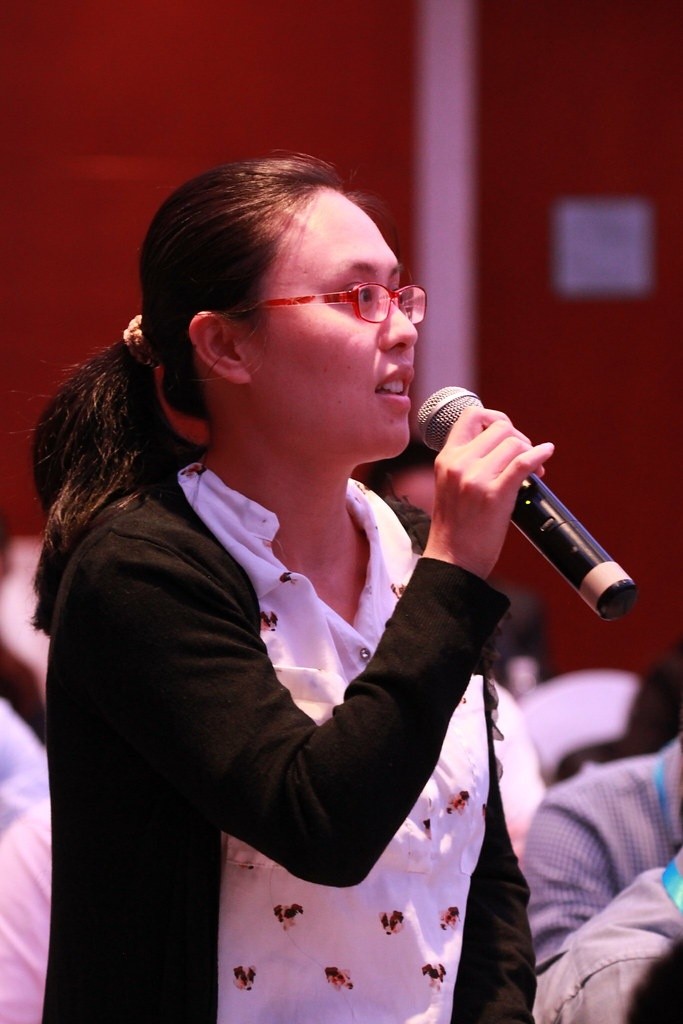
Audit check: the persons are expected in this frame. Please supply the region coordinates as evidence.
[35,153,555,1023]
[0,440,683,1024]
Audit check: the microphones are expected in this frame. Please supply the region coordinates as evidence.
[417,386,637,620]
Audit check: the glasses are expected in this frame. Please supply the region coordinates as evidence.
[228,280,428,324]
